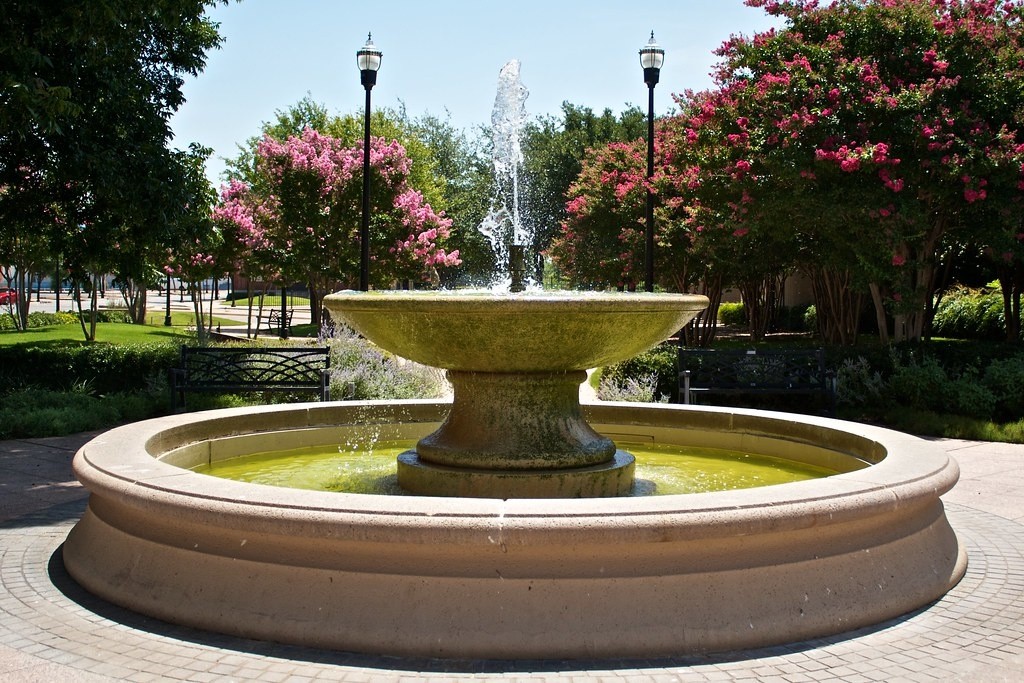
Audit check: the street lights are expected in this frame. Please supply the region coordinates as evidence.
[638,30,666,292]
[357,30,382,290]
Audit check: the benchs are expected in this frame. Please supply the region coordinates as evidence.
[256,309,295,337]
[677,348,833,417]
[168,342,331,412]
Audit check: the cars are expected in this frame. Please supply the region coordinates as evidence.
[0,287,26,305]
